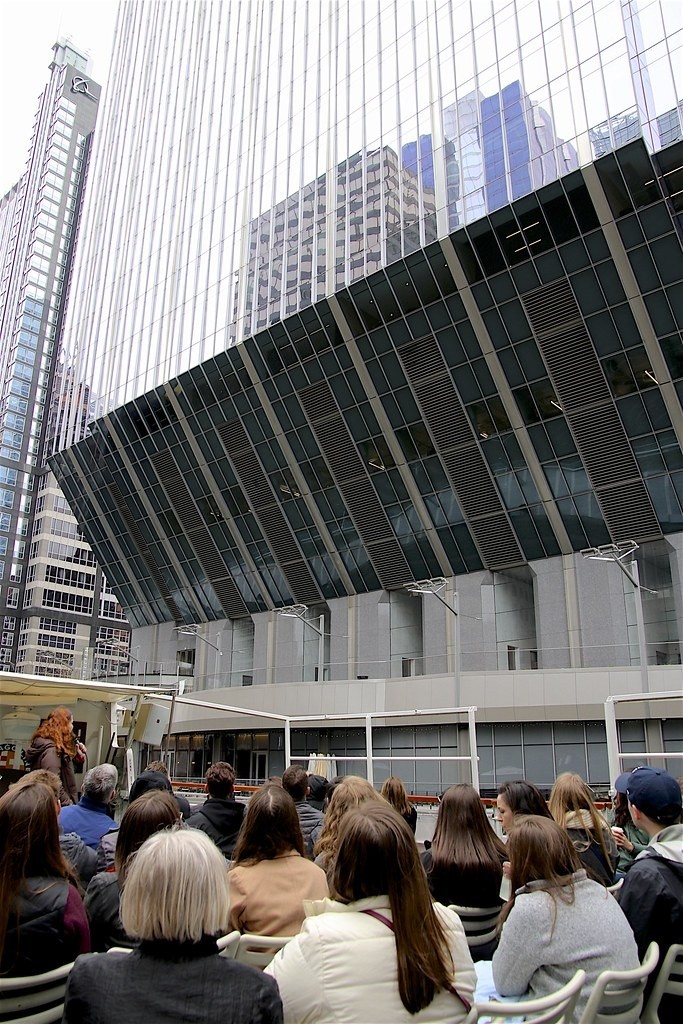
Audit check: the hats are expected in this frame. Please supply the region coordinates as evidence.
[615,773,632,794]
[627,766,682,821]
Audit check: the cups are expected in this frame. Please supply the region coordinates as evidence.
[610,826,624,848]
[499,862,511,902]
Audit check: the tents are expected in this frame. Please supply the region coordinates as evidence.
[0,671,178,792]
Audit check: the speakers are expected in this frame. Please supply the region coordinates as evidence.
[134,703,170,746]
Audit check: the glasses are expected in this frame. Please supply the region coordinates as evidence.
[437,795,442,804]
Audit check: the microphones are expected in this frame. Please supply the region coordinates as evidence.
[71,734,86,754]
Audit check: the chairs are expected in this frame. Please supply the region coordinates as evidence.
[447,878,683,1024]
[0,931,294,1024]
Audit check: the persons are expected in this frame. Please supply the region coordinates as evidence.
[95,770,190,875]
[282,765,351,863]
[61,825,284,1024]
[419,783,511,963]
[609,771,649,876]
[143,761,191,824]
[58,763,120,851]
[495,779,556,881]
[0,780,89,1024]
[226,783,331,970]
[474,815,643,1024]
[379,776,417,836]
[546,772,620,895]
[16,769,98,902]
[184,762,247,863]
[312,775,393,875]
[614,765,683,1024]
[263,802,478,1024]
[25,706,87,808]
[262,777,283,789]
